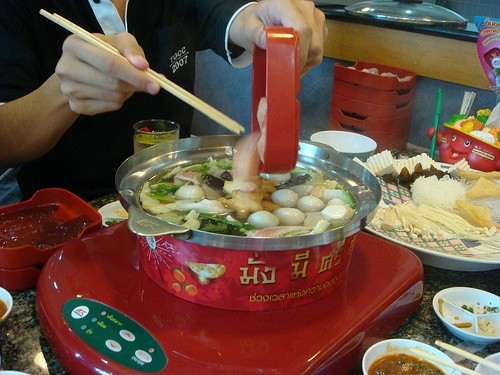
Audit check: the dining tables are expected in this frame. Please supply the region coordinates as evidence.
[0,126,500,375]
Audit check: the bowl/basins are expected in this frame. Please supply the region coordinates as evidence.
[310,130,378,162]
[0,287,13,325]
[474,352,500,375]
[432,287,500,344]
[362,339,462,375]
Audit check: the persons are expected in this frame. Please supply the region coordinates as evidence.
[0,0,329,202]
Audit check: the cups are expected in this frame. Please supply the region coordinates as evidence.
[134,119,180,154]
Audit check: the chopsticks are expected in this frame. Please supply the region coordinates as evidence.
[410,340,500,375]
[39,8,245,134]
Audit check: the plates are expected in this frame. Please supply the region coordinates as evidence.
[98,199,128,227]
[0,188,103,292]
[252,26,301,174]
[326,60,416,155]
[364,161,500,271]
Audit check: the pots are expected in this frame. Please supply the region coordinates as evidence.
[114,134,381,313]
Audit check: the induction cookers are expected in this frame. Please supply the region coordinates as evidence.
[37,219,424,375]
